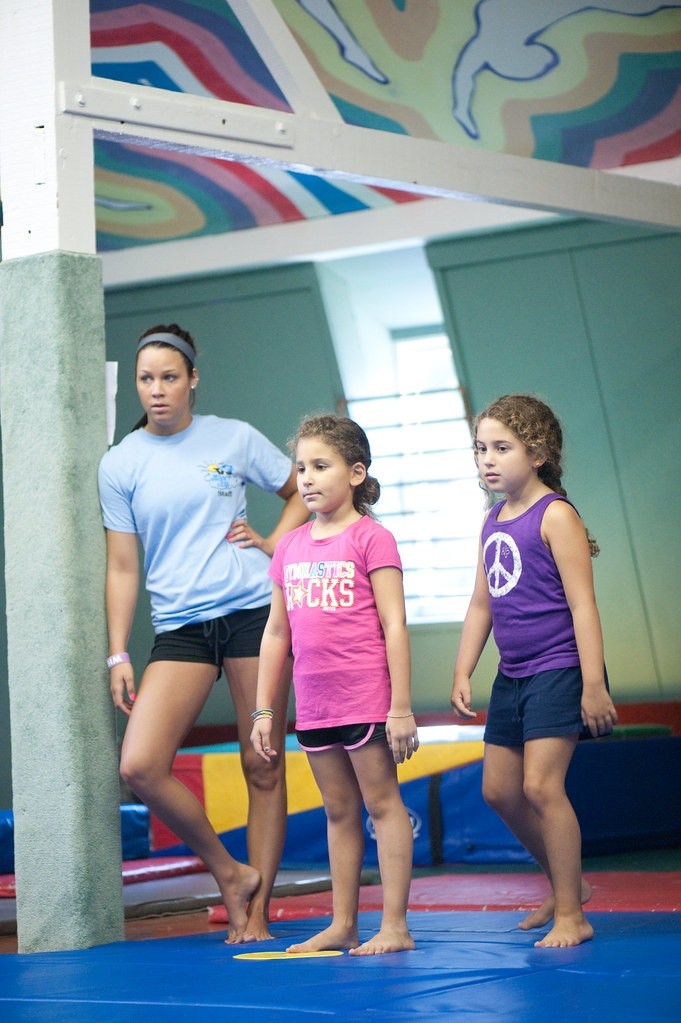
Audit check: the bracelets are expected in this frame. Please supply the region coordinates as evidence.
[387,712,413,718]
[250,709,274,723]
[106,652,132,669]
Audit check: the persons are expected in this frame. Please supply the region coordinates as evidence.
[97,324,310,943]
[248,412,420,955]
[451,396,618,950]
